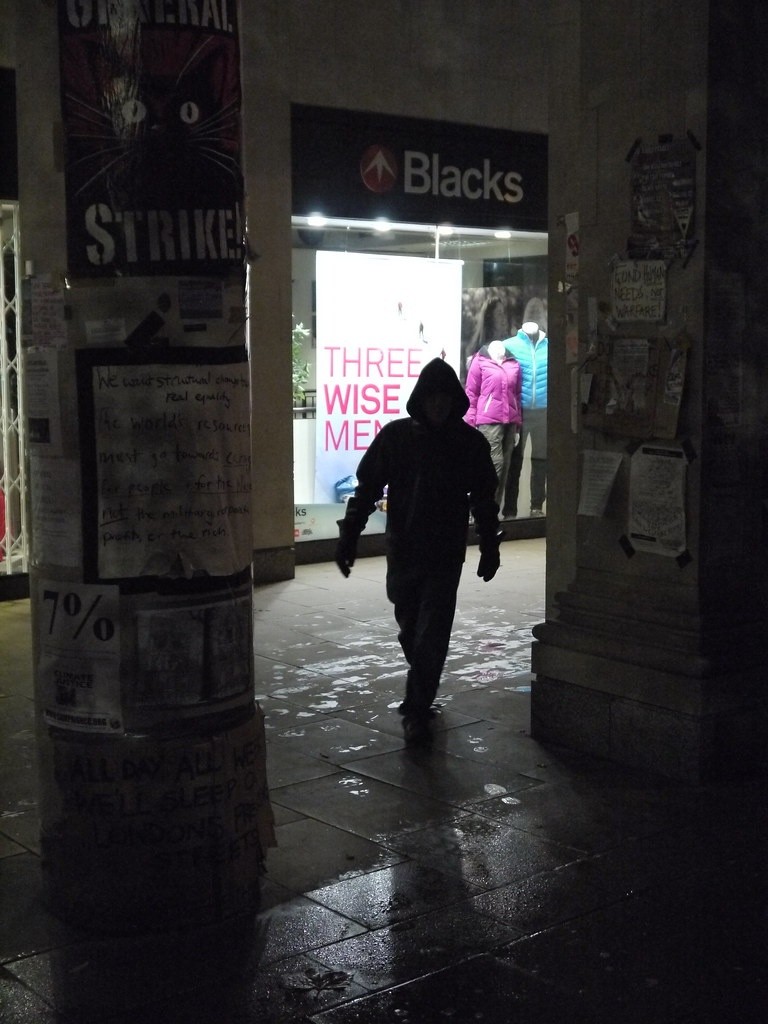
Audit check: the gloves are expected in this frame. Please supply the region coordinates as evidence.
[477,538,500,581]
[334,522,360,577]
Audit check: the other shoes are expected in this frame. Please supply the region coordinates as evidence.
[402,714,431,748]
[503,515,517,521]
[399,704,435,718]
[531,509,545,517]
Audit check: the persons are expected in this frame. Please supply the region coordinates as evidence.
[333,357,501,742]
[464,340,522,525]
[468,296,505,355]
[466,322,549,520]
